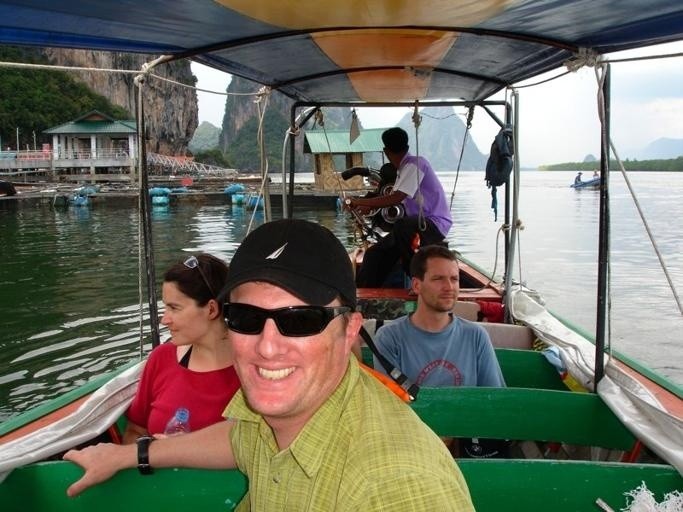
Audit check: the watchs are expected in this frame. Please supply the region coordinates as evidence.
[134,434,158,475]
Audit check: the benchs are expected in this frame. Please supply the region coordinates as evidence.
[0,299,683,512]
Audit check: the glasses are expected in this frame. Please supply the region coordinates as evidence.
[183,255,217,299]
[222,303,352,337]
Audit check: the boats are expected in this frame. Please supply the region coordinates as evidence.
[568,173,611,193]
[1,167,683,508]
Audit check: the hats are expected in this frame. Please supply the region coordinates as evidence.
[218,218,356,312]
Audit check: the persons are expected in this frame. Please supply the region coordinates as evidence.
[61,218,502,512]
[574,171,584,186]
[370,246,509,448]
[357,220,421,289]
[592,170,600,181]
[342,126,453,255]
[118,251,243,449]
